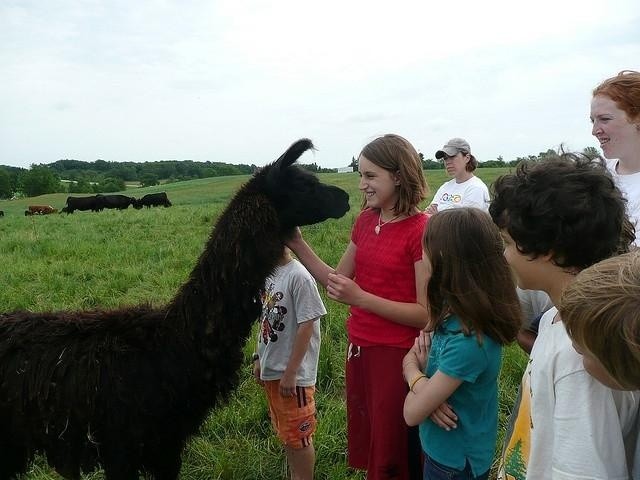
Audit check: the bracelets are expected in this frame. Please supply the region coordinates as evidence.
[409,374,426,391]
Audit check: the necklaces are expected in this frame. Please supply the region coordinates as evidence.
[375,212,403,236]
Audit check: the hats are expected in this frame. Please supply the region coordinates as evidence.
[435,137,471,159]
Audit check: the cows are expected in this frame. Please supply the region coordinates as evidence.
[25,192,172,217]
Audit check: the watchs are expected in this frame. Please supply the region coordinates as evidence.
[252,353,259,361]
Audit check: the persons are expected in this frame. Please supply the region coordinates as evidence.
[252,244,327,480]
[484,141,640,480]
[515,288,555,354]
[424,137,491,218]
[284,134,431,480]
[557,248,640,392]
[402,206,522,480]
[590,69,640,247]
